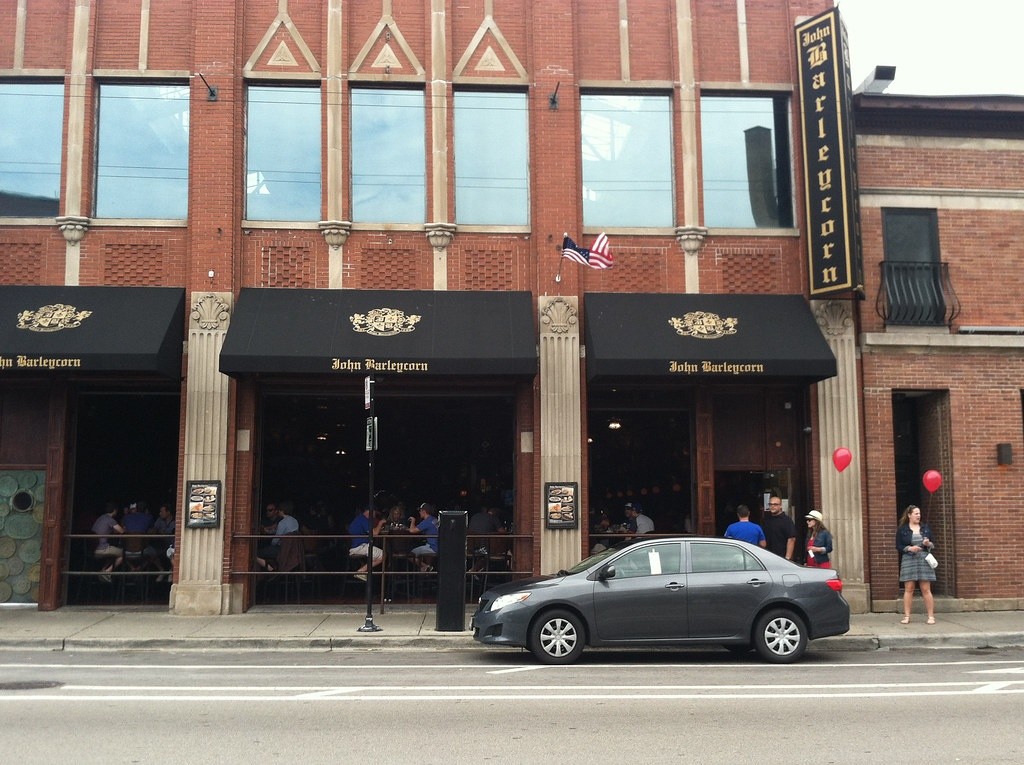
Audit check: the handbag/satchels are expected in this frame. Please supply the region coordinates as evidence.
[925,547,938,569]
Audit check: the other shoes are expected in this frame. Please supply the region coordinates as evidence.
[268,574,278,582]
[155,574,166,582]
[168,575,173,582]
[468,567,483,580]
[900,617,910,624]
[422,565,434,572]
[99,574,112,583]
[928,616,935,624]
[353,574,367,581]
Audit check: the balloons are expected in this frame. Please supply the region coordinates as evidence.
[833,447,852,473]
[923,470,941,492]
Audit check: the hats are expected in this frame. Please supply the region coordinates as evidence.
[624,503,632,507]
[631,503,641,511]
[417,502,431,513]
[805,510,823,522]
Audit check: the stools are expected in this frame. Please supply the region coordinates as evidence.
[418,528,513,601]
[72,532,117,603]
[302,529,328,599]
[331,531,377,600]
[386,530,417,601]
[120,530,150,605]
[262,531,307,603]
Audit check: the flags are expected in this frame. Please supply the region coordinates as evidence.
[561,231,614,271]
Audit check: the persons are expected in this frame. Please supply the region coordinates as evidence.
[468,506,513,582]
[256,500,299,571]
[590,502,654,556]
[895,505,938,624]
[805,510,833,569]
[761,497,796,560]
[724,505,767,548]
[345,503,439,582]
[91,502,175,582]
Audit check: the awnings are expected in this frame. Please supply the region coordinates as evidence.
[583,293,837,385]
[219,287,539,382]
[0,285,186,382]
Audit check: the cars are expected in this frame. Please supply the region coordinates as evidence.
[468,537,851,665]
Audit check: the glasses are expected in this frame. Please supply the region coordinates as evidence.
[768,503,781,507]
[908,505,916,512]
[265,508,276,512]
[624,506,631,510]
[807,518,814,522]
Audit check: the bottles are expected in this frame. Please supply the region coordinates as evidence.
[390,522,402,532]
[505,520,512,532]
